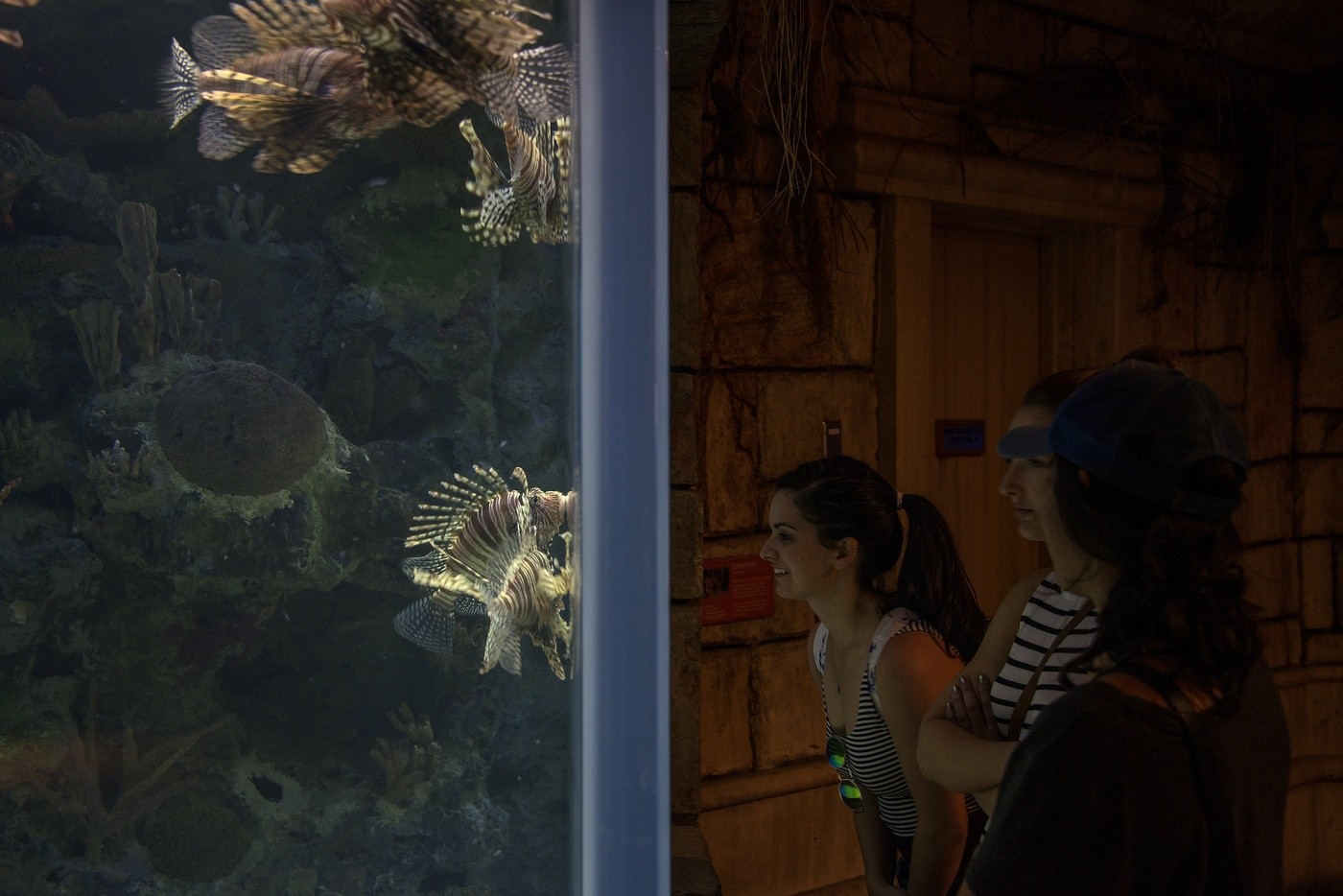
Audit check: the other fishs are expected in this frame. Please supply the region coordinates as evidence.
[476,528,572,681]
[155,0,572,246]
[392,464,566,658]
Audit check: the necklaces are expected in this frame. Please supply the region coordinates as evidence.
[828,591,877,695]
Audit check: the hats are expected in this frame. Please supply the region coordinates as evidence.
[996,357,1252,519]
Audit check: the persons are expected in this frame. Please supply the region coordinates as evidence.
[759,454,991,896]
[917,360,1118,822]
[958,355,1292,896]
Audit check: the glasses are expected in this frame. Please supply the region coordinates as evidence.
[826,735,866,814]
